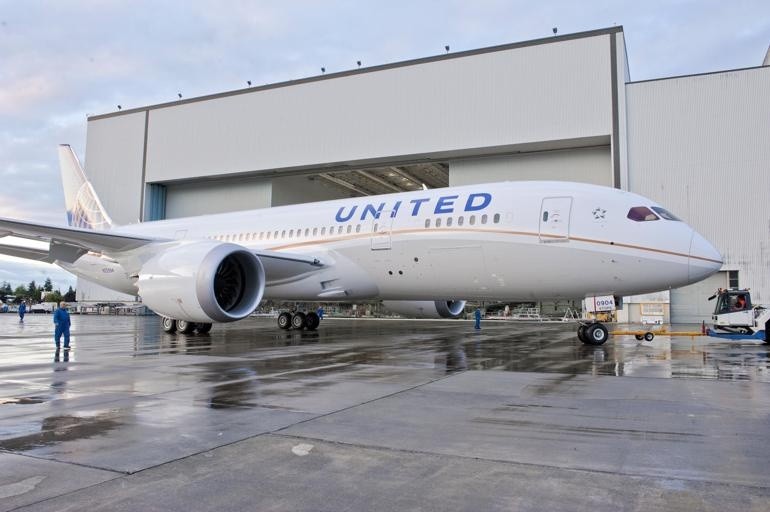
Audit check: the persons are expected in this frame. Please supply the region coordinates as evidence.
[317,306,324,320]
[18,299,25,321]
[731,295,746,311]
[49,350,69,393]
[475,305,481,328]
[54,301,71,348]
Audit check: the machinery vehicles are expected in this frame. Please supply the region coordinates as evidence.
[705,285,770,343]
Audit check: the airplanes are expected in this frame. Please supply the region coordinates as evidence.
[0,139,731,348]
